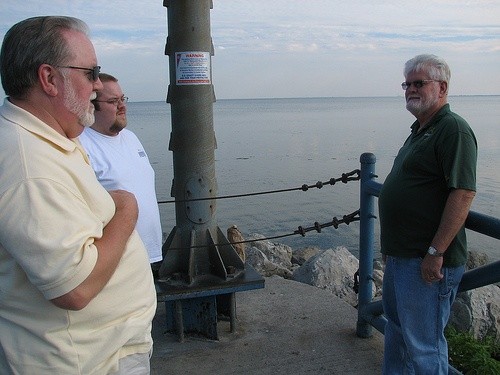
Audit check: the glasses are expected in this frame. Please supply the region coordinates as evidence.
[401,80,441,90]
[97,96,128,105]
[57,66,101,81]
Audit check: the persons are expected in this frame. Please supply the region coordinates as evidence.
[0,15,157,375]
[379,54,479,375]
[77,73,163,282]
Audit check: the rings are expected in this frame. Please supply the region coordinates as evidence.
[426,273,439,281]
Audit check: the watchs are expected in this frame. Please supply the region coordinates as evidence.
[427,246,445,258]
